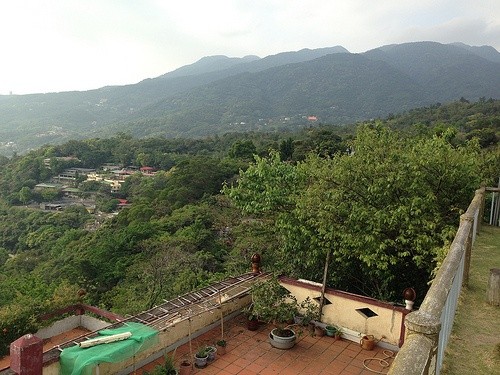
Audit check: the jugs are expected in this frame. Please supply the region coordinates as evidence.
[360,335,387,351]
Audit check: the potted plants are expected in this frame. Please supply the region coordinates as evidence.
[242,274,325,349]
[142,337,226,375]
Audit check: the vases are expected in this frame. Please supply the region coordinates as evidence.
[307,322,338,337]
